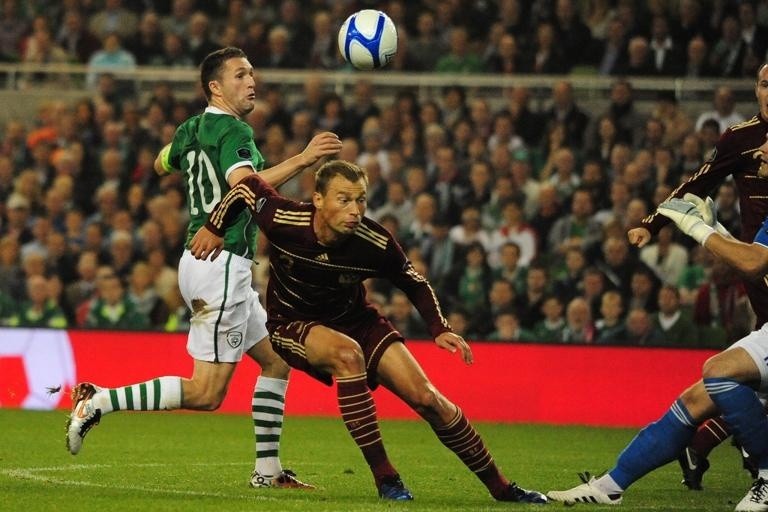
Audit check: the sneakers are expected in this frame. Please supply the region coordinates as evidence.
[495,472,623,505]
[377,474,413,500]
[249,470,315,489]
[680,446,709,490]
[66,383,101,455]
[735,444,768,512]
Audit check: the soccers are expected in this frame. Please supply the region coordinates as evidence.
[337,9,397,68]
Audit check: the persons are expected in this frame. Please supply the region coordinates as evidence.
[630,64,768,488]
[65,46,344,491]
[190,161,546,504]
[548,191,767,510]
[0,0,767,351]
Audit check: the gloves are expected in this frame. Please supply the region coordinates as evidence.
[657,192,730,247]
